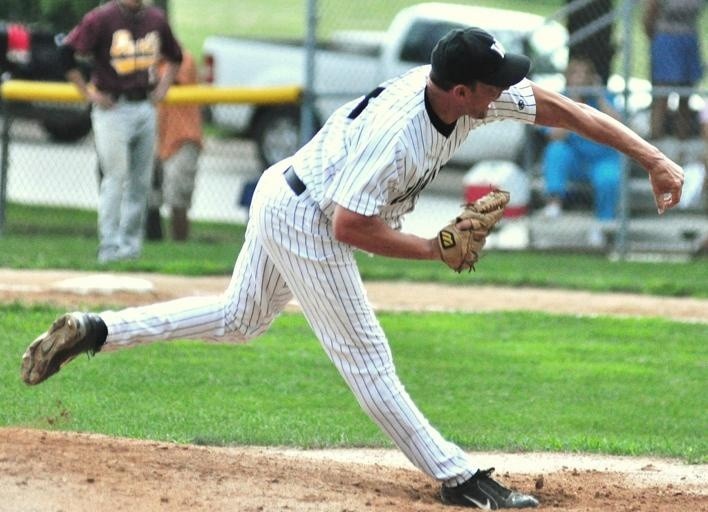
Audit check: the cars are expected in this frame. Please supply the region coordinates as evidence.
[0,0,171,143]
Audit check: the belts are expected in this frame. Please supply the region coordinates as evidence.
[283,167,307,196]
[109,92,146,101]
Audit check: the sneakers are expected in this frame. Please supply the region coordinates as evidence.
[97,247,142,262]
[21,312,106,385]
[534,206,561,218]
[439,470,537,510]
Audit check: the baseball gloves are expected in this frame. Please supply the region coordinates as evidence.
[438,189,510,272]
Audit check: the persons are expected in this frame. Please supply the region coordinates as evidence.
[535,56,629,223]
[18,23,688,510]
[56,2,183,263]
[145,50,204,244]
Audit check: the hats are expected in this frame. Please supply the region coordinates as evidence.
[431,27,531,88]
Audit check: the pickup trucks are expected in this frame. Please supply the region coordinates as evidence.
[198,2,707,169]
